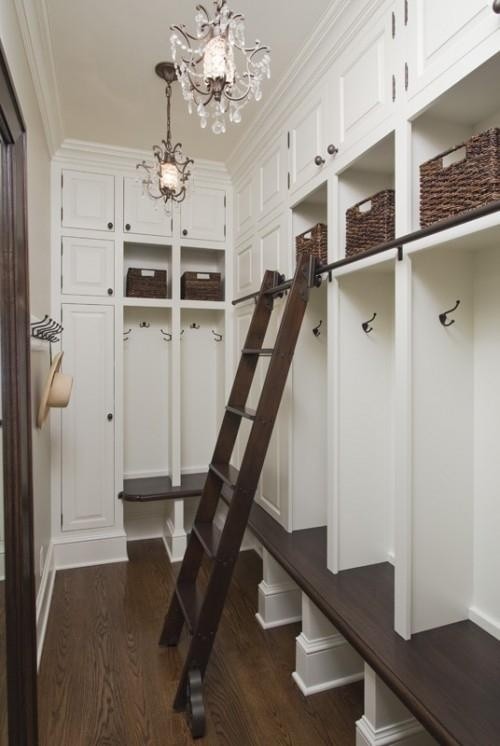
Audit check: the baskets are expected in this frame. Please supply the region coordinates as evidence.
[126,267,221,301]
[296,125,500,272]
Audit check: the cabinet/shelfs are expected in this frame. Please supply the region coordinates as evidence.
[228,0,499,745]
[52,160,228,572]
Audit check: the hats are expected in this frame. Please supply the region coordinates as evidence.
[37,351,73,430]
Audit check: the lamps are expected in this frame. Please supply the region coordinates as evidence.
[168,0,272,136]
[135,62,194,216]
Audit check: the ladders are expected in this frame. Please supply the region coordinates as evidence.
[158,250,321,738]
